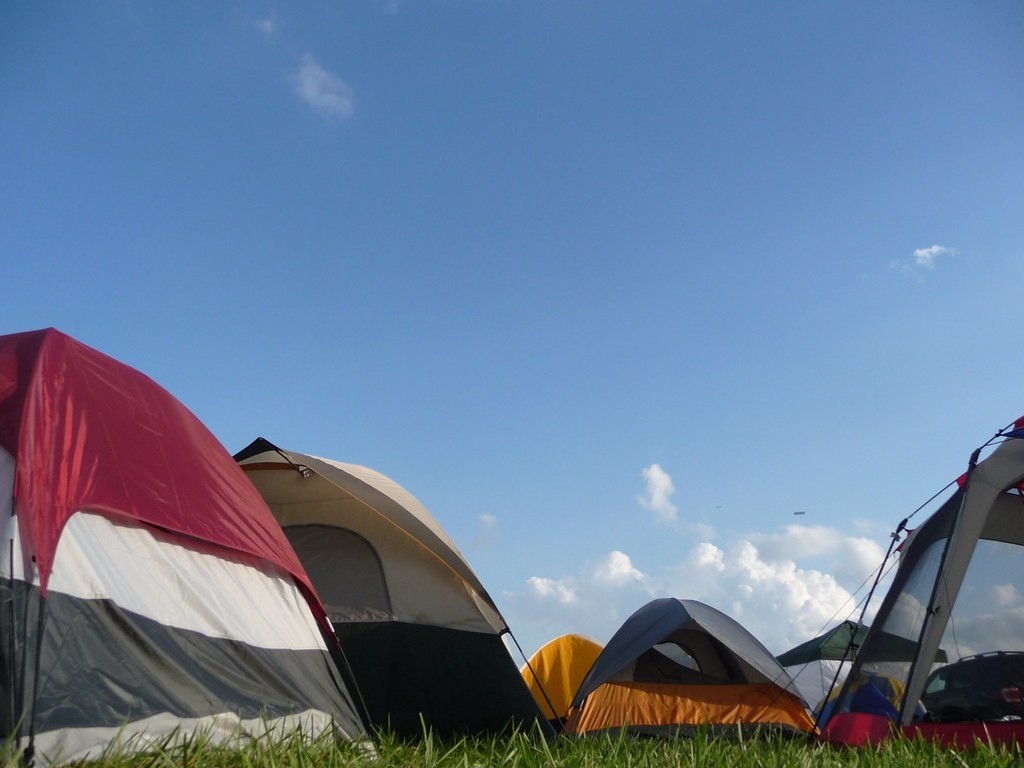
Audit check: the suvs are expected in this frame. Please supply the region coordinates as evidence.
[920,650,1024,721]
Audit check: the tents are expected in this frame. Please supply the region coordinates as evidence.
[560,597,822,748]
[234,435,567,746]
[816,408,1024,750]
[775,618,948,715]
[519,632,607,735]
[0,324,380,768]
[805,671,927,746]
